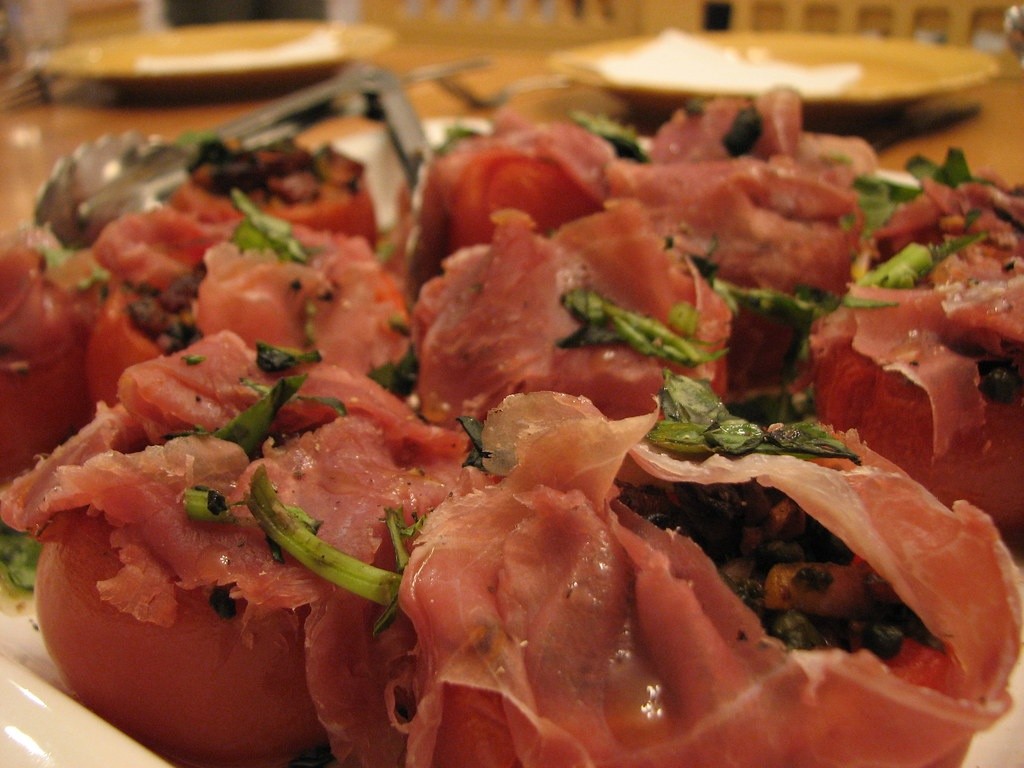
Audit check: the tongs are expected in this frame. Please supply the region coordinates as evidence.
[36,47,436,263]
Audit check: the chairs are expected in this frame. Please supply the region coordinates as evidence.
[727,0,1011,45]
[361,0,704,52]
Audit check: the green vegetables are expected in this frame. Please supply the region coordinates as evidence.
[0,103,1024,674]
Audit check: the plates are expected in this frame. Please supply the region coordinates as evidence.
[0,117,1024,768]
[548,26,1001,138]
[27,19,400,97]
